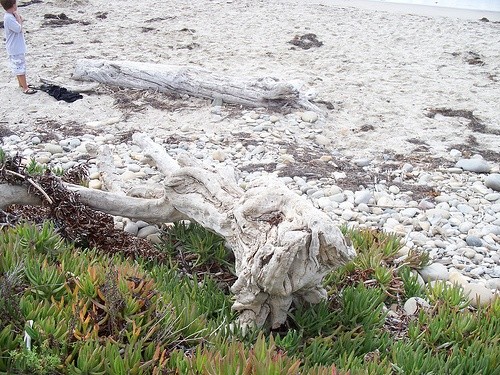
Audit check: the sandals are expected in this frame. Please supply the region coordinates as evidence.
[24,82,34,88]
[23,88,37,94]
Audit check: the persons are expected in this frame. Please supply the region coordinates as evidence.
[0,0,38,94]
